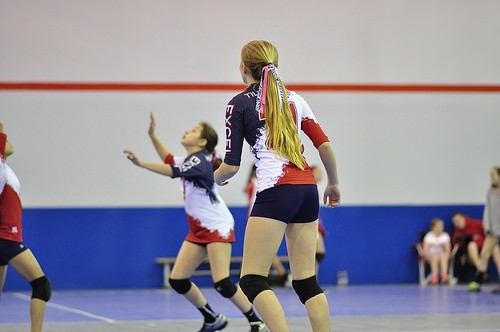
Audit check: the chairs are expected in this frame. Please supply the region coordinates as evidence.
[412,230,458,289]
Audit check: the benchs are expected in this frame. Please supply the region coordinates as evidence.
[156,257,289,288]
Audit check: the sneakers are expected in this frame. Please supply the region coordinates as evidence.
[250,322,268,332]
[199,314,228,332]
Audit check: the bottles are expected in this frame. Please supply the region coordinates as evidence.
[336,271,349,287]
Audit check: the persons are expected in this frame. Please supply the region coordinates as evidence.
[447,211,485,282]
[214,39,341,332]
[309,165,329,276]
[467,165,500,294]
[421,217,453,283]
[245,163,291,286]
[122,113,268,332]
[0,123,53,332]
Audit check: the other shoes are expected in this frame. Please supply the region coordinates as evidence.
[440,273,448,283]
[433,274,439,284]
[492,285,500,293]
[467,282,482,291]
